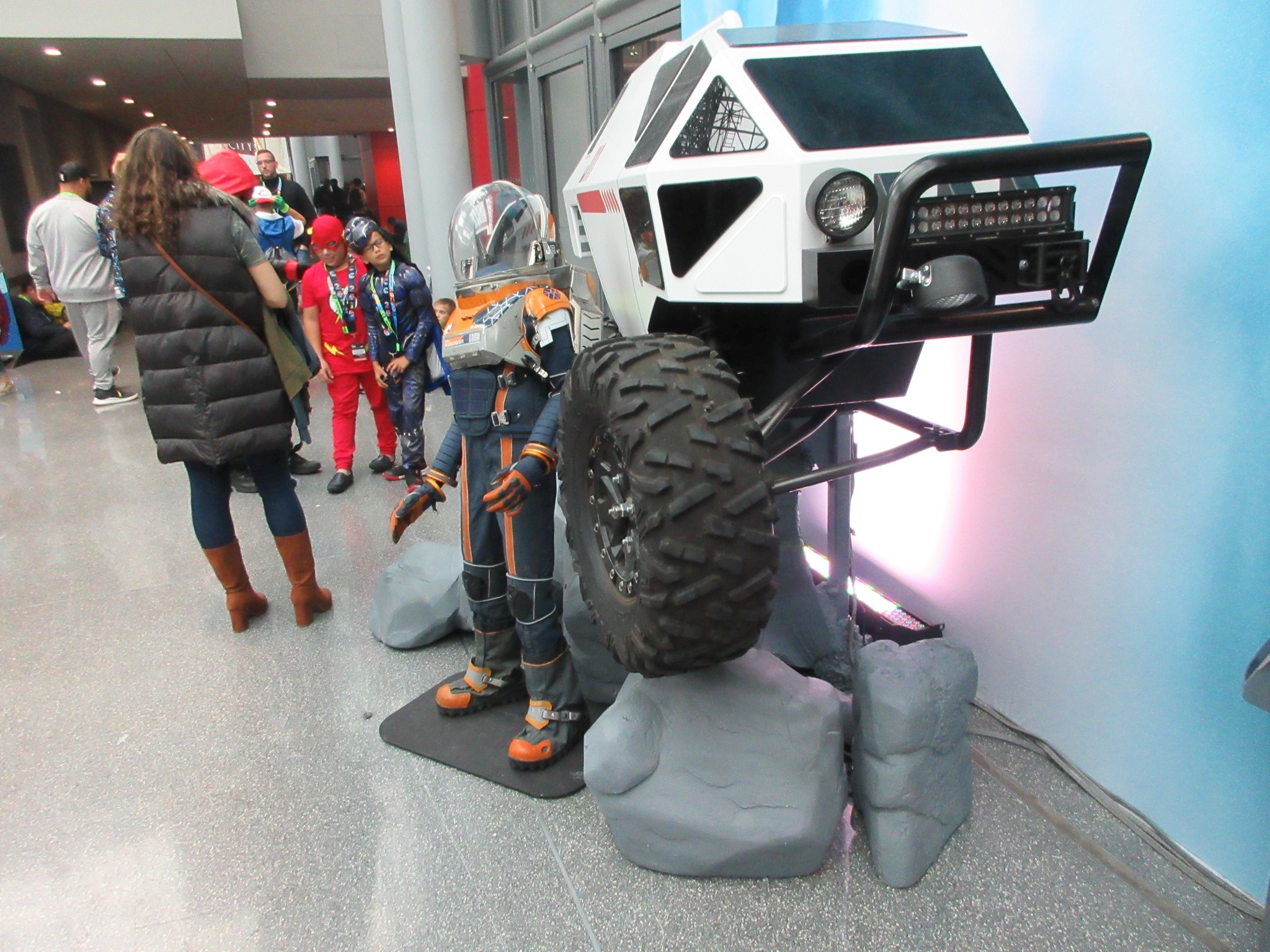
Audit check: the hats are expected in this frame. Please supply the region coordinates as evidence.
[58,161,98,183]
[250,185,276,205]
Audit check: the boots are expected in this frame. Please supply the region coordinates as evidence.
[435,626,528,718]
[202,540,269,633]
[273,526,333,628]
[507,643,593,772]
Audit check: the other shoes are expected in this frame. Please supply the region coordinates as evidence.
[369,454,396,473]
[289,452,322,475]
[230,470,259,493]
[0,380,17,400]
[327,470,354,495]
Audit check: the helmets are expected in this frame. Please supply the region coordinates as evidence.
[446,179,576,320]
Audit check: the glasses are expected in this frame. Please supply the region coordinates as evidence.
[361,238,386,254]
[255,159,274,165]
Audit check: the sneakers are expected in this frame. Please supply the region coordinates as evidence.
[405,467,424,493]
[382,458,430,481]
[112,366,120,378]
[91,386,139,406]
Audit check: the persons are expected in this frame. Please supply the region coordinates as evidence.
[300,216,457,495]
[0,152,140,407]
[389,180,590,771]
[114,124,333,633]
[313,177,407,244]
[196,149,322,494]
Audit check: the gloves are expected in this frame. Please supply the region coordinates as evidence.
[482,455,547,518]
[388,477,447,544]
[272,195,290,213]
